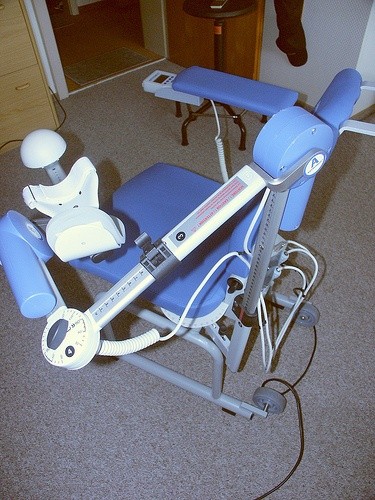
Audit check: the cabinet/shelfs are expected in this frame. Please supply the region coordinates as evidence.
[0,0,61,159]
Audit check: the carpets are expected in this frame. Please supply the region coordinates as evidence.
[63,45,152,87]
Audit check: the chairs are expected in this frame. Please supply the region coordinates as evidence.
[0,64,366,423]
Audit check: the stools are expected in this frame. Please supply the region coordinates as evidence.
[175,0,258,151]
